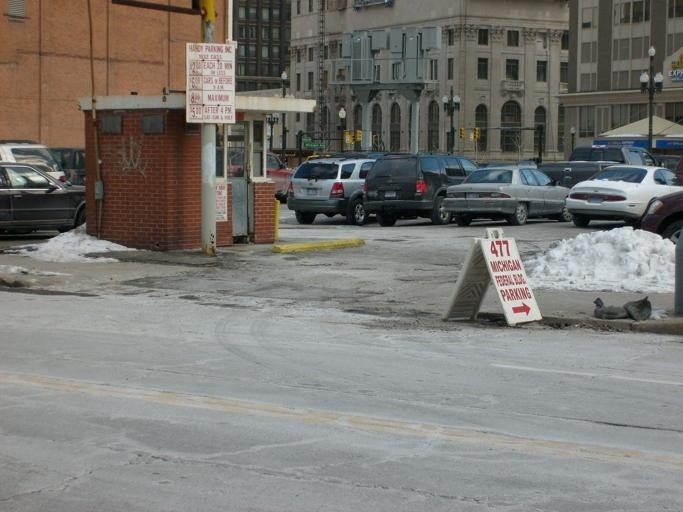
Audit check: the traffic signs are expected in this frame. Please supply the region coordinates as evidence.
[479,228,543,326]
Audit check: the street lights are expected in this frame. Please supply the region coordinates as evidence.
[281,71,287,149]
[640,46,664,153]
[266,113,279,151]
[339,108,346,152]
[442,86,460,153]
[570,127,576,151]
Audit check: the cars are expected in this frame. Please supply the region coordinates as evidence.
[0,143,85,237]
[216,147,295,203]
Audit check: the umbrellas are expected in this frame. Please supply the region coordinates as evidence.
[597,116,683,148]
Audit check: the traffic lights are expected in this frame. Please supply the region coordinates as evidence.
[373,135,377,145]
[351,135,355,144]
[357,130,362,141]
[460,128,464,139]
[474,128,480,140]
